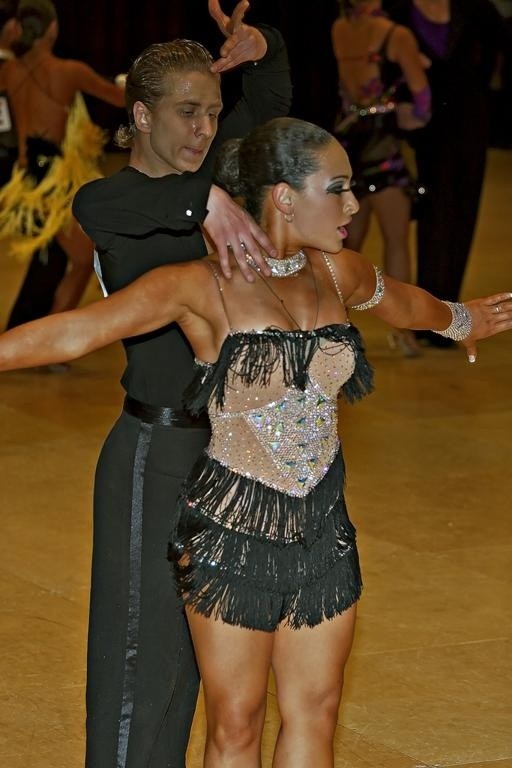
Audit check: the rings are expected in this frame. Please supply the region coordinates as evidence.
[496,306,500,312]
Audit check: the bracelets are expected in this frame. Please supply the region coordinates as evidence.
[433,301,473,341]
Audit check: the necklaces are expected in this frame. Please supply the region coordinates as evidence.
[243,252,306,277]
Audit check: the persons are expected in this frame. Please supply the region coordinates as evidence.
[382,1,512,349]
[68,36,276,768]
[1,115,511,767]
[0,1,130,333]
[293,1,429,360]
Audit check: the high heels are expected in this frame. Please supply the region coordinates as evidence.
[381,319,425,360]
